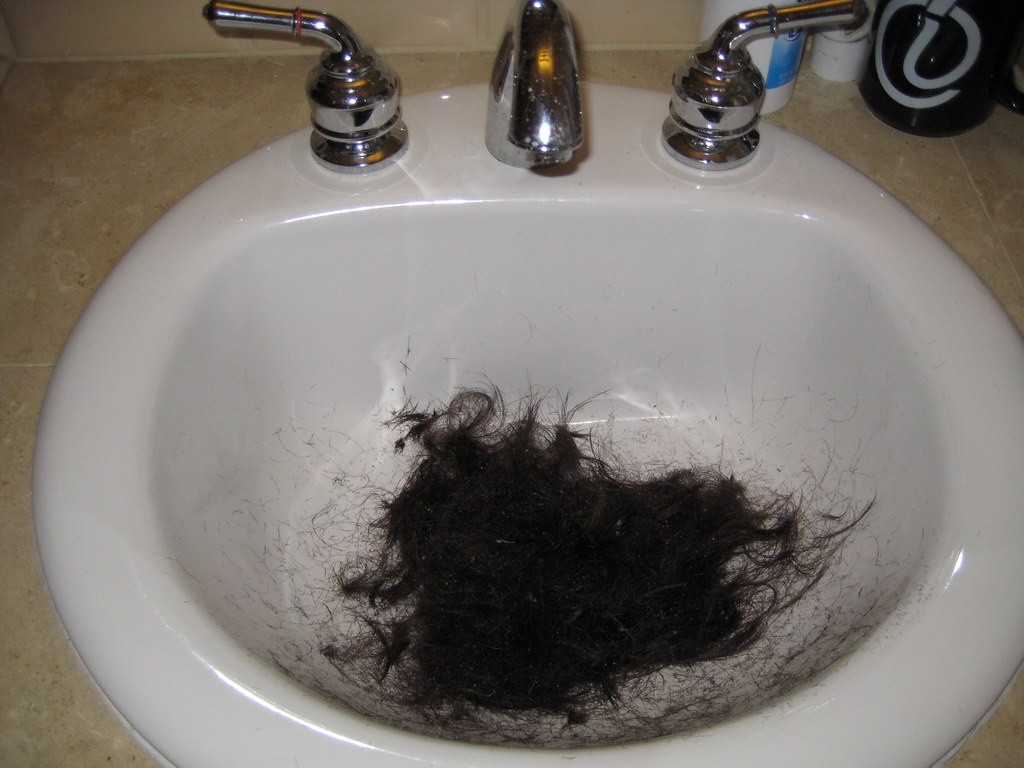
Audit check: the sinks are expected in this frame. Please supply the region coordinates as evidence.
[32,83,1024,768]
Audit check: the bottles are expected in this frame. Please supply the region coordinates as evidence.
[697,0,1024,140]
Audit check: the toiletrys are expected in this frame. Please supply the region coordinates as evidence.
[809,0,883,84]
[700,0,811,116]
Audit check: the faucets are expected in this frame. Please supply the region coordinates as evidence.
[481,1,586,172]
